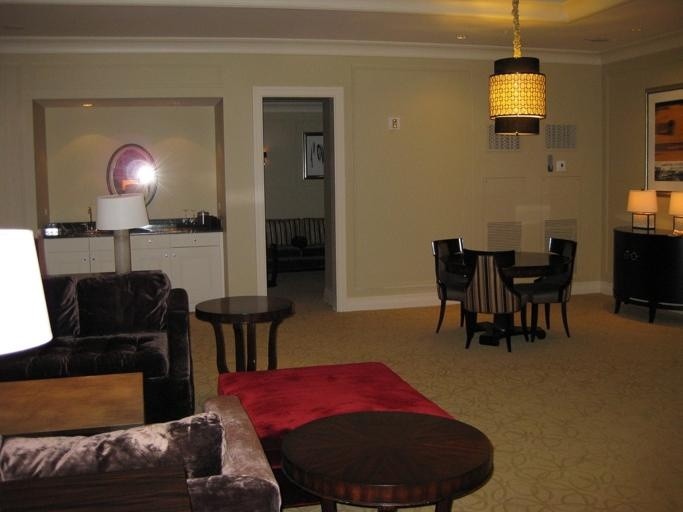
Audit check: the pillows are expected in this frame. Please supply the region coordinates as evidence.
[0,410,222,482]
[42,271,171,336]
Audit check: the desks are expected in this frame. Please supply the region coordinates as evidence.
[612,225,683,323]
[0,371,146,436]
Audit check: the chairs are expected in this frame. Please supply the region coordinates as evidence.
[0,395,282,510]
[431,235,577,352]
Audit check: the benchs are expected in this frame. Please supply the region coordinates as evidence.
[266,217,326,287]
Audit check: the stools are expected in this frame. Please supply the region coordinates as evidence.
[217,362,457,508]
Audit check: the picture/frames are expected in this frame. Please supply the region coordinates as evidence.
[644,83,683,196]
[302,132,325,181]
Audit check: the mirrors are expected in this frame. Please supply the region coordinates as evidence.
[105,143,158,206]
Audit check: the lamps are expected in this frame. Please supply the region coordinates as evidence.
[96,192,151,274]
[626,190,659,234]
[0,228,55,357]
[488,0,547,136]
[668,191,683,230]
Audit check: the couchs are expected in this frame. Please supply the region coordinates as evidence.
[0,269,195,420]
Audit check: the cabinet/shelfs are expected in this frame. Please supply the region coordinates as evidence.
[43,232,115,276]
[129,232,225,313]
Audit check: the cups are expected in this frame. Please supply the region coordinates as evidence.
[197,212,209,227]
